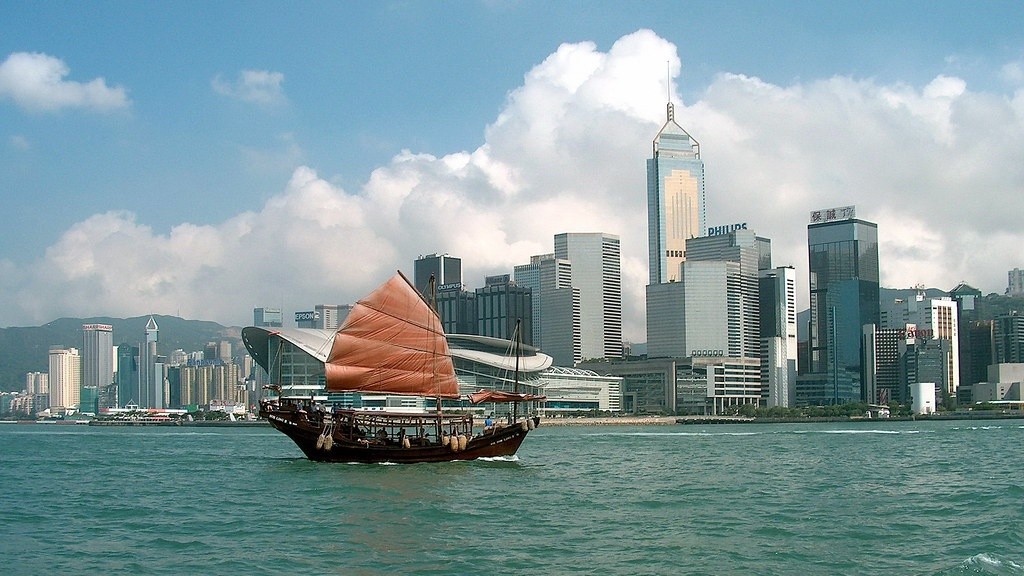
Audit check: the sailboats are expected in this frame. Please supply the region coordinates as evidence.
[258,269,540,462]
[466,318,546,427]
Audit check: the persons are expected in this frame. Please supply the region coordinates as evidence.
[483,415,493,427]
[263,399,429,449]
